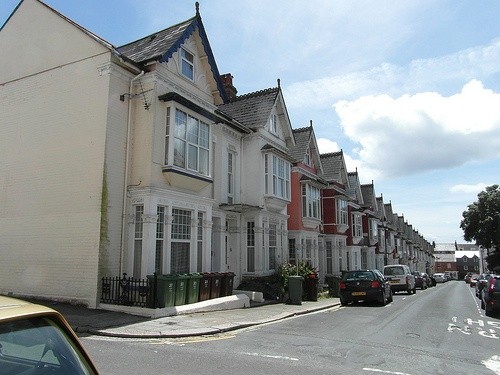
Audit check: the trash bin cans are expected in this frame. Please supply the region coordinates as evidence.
[289,276,302,305]
[198,272,211,300]
[147,274,174,307]
[221,271,236,295]
[186,272,199,303]
[211,271,221,298]
[327,274,341,297]
[305,273,319,301]
[175,272,187,305]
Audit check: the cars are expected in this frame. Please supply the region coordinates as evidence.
[0,295,101,375]
[464,272,500,317]
[339,269,395,306]
[412,270,452,290]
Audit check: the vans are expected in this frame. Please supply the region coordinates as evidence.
[383,264,416,295]
